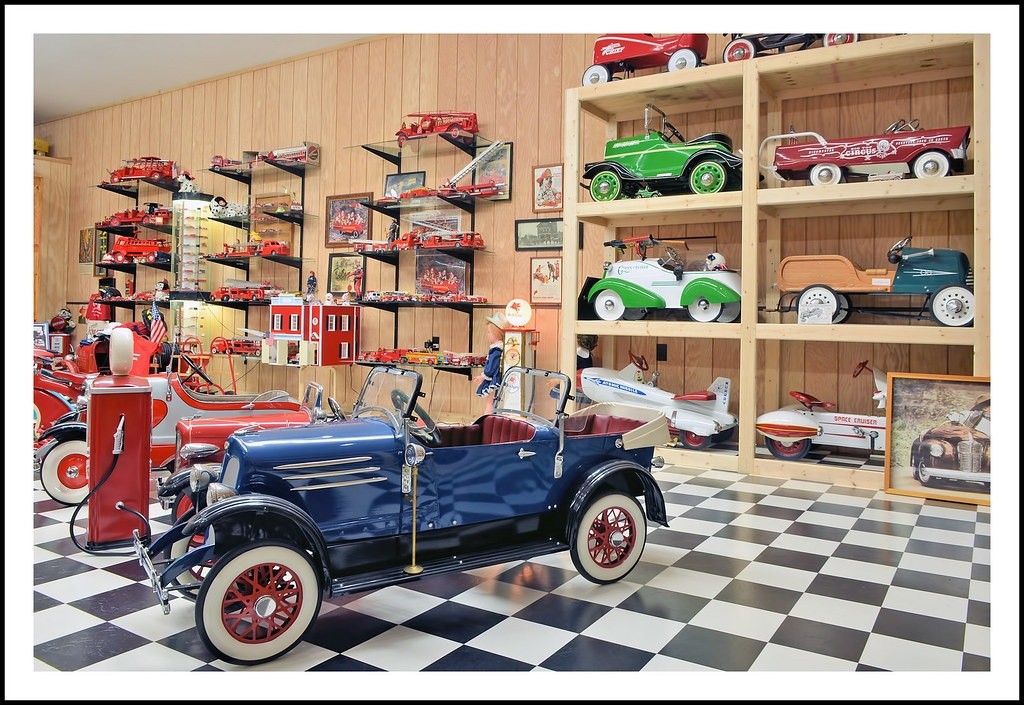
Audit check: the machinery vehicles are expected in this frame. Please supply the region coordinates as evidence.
[442,352,460,365]
[381,349,417,362]
[211,337,264,356]
[217,237,289,257]
[357,348,372,361]
[367,345,386,363]
[210,278,280,302]
[400,137,505,205]
[457,355,487,367]
[354,214,486,251]
[396,111,480,152]
[111,155,178,183]
[107,202,171,227]
[361,289,486,305]
[110,232,171,261]
[397,350,442,366]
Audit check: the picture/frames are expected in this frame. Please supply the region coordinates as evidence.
[325,192,373,248]
[93,222,114,277]
[472,141,513,201]
[532,164,564,213]
[884,372,991,506]
[384,171,426,199]
[515,218,563,250]
[254,192,295,257]
[34,322,50,351]
[327,252,366,297]
[530,256,563,304]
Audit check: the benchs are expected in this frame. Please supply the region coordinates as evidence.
[553,414,648,431]
[481,415,534,444]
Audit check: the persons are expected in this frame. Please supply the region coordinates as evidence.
[347,264,364,301]
[474,311,505,413]
[387,218,397,249]
[537,260,559,281]
[303,271,317,302]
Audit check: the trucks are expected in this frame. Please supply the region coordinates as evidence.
[209,156,243,176]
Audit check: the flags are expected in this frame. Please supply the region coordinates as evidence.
[150,301,167,348]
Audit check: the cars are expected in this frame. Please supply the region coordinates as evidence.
[577,33,973,333]
[911,393,991,491]
[564,347,740,452]
[755,358,893,461]
[33,323,672,667]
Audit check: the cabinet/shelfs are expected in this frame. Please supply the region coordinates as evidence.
[195,148,318,360]
[89,175,179,321]
[562,34,993,419]
[343,128,493,381]
[34,156,71,322]
